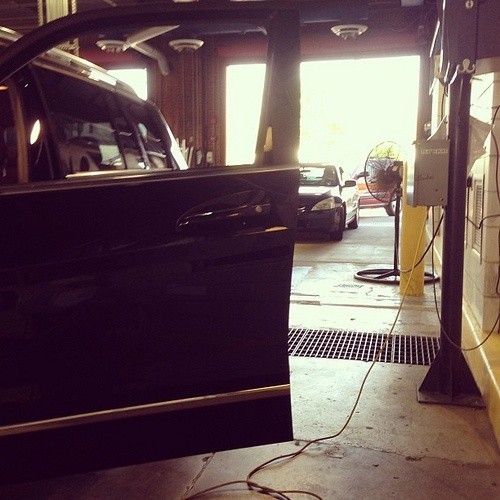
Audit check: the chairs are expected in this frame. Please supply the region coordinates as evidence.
[68,137,116,174]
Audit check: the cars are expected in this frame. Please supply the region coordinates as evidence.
[353,171,404,216]
[298,160,360,243]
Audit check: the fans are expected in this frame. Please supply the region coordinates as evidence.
[354,142,440,285]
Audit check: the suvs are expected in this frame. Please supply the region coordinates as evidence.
[0,3,301,485]
